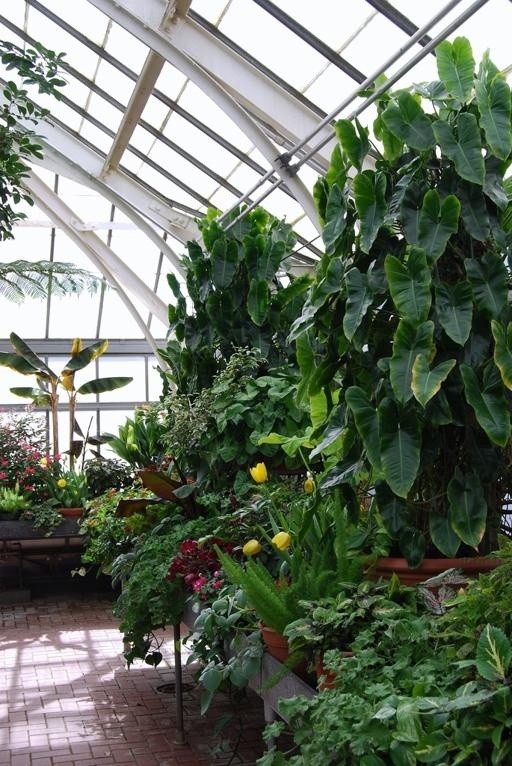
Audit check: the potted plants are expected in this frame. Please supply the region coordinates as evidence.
[292,24,512,591]
[284,577,416,693]
[218,549,317,678]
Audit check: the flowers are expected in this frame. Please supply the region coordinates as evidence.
[250,462,268,483]
[169,537,233,593]
[0,412,140,537]
[243,539,261,555]
[272,529,291,549]
